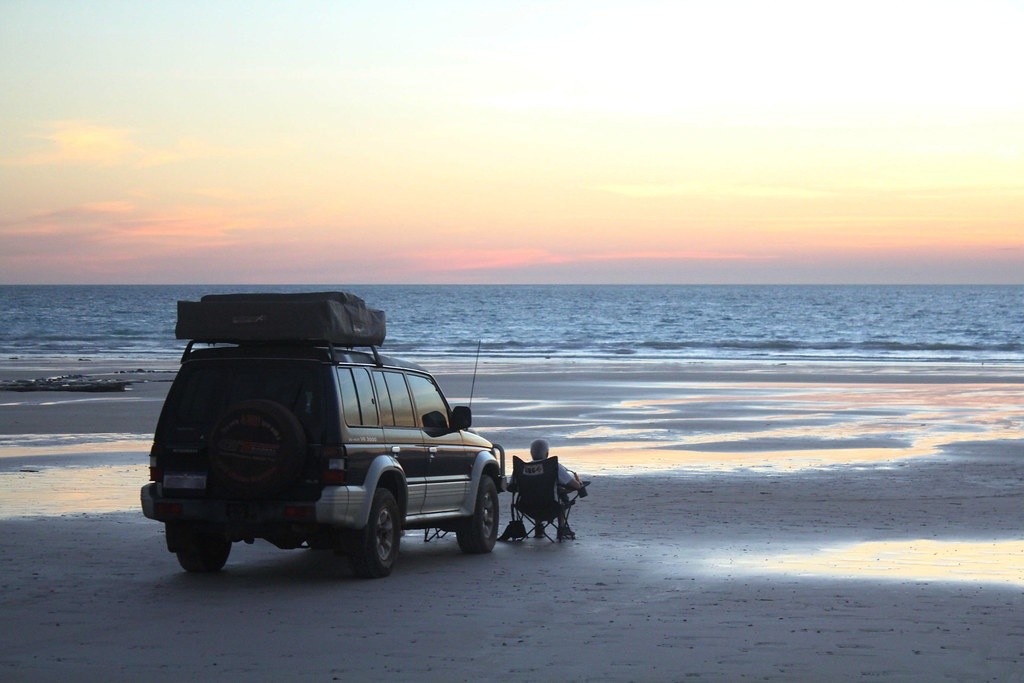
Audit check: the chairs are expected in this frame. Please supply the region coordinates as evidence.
[510,455,591,543]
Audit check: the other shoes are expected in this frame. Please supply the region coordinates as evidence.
[535,525,544,537]
[556,527,576,538]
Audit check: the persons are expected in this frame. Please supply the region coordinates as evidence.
[508,439,580,539]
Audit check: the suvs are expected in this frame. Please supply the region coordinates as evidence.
[139,339,507,577]
